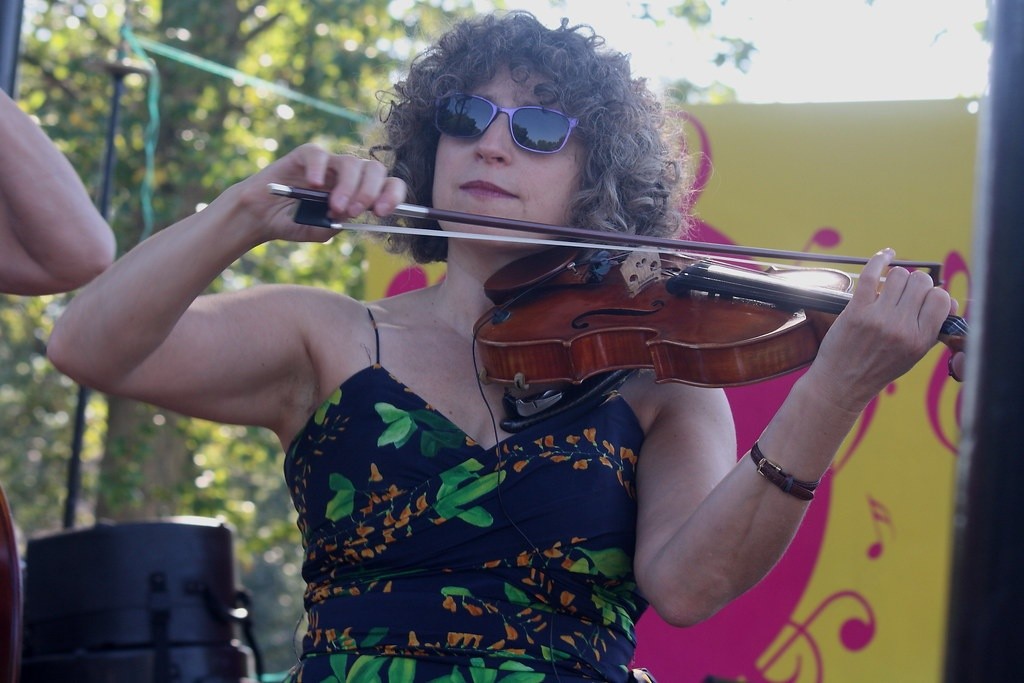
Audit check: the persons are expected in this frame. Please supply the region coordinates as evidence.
[47,9,958,683]
[0,87,116,297]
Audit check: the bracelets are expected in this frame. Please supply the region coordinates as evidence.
[750,438,822,500]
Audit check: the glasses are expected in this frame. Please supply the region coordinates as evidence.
[432,91,579,154]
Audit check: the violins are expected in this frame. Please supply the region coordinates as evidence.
[471,237,967,388]
